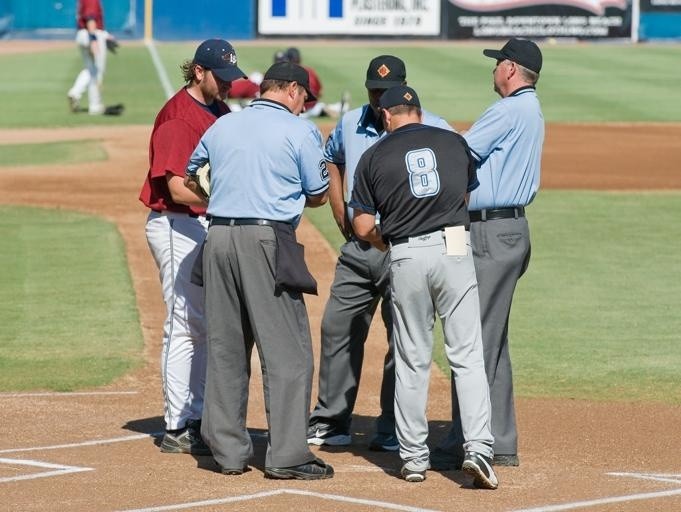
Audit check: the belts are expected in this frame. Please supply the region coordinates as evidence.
[469,207,526,222]
[391,238,409,246]
[149,208,206,218]
[208,216,271,226]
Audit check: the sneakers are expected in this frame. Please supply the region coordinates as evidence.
[461,451,498,489]
[455,455,519,470]
[368,432,399,452]
[211,457,241,475]
[264,458,334,480]
[161,417,202,433]
[399,468,426,482]
[160,429,212,456]
[307,419,353,446]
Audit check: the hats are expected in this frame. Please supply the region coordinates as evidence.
[483,37,543,72]
[263,62,318,102]
[364,55,407,92]
[373,87,421,121]
[193,39,248,82]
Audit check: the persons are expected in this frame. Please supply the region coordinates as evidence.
[138,38,248,457]
[433,37,546,466]
[274,52,287,66]
[67,0,118,118]
[184,62,335,481]
[286,48,349,119]
[347,86,499,489]
[224,72,266,100]
[305,55,460,452]
